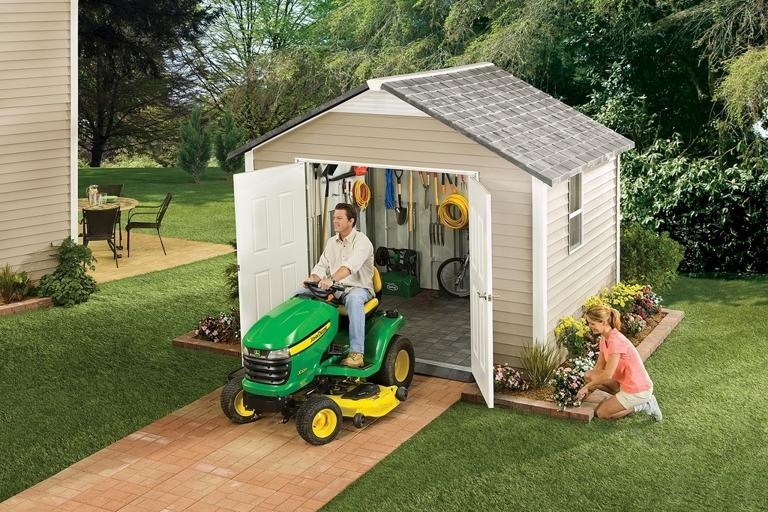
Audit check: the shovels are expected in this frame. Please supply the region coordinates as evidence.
[321,164,338,256]
[394,169,407,225]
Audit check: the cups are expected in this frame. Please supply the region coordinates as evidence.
[87,185,107,207]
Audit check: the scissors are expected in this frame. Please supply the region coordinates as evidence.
[419,171,430,210]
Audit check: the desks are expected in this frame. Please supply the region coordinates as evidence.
[78,196,139,259]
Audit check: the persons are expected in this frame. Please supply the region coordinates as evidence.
[575,305,662,422]
[299,202,377,368]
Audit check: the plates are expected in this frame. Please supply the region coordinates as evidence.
[105,196,117,202]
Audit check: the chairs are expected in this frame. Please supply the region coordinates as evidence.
[81,184,173,271]
[333,265,383,319]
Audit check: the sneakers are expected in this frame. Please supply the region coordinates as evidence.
[646,394,663,422]
[339,351,365,368]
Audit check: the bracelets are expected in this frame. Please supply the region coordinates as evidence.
[330,276,335,285]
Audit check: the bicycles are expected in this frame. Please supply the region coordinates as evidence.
[437,254,470,298]
